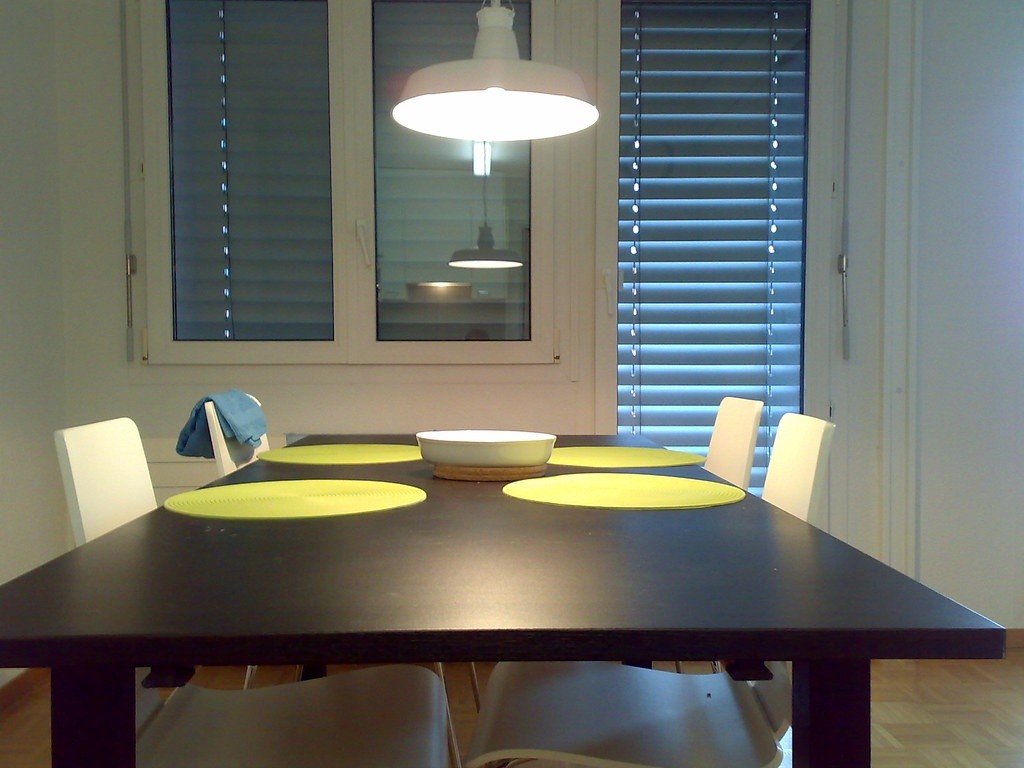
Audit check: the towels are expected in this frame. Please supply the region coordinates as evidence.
[174,383,267,456]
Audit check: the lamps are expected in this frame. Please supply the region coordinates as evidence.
[392,0,598,141]
[449,139,523,270]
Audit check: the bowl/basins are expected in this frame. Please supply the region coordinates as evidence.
[416,431,556,467]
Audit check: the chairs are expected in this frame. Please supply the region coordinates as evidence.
[54,393,463,768]
[467,395,836,768]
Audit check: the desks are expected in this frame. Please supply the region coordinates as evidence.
[1,436,1006,768]
